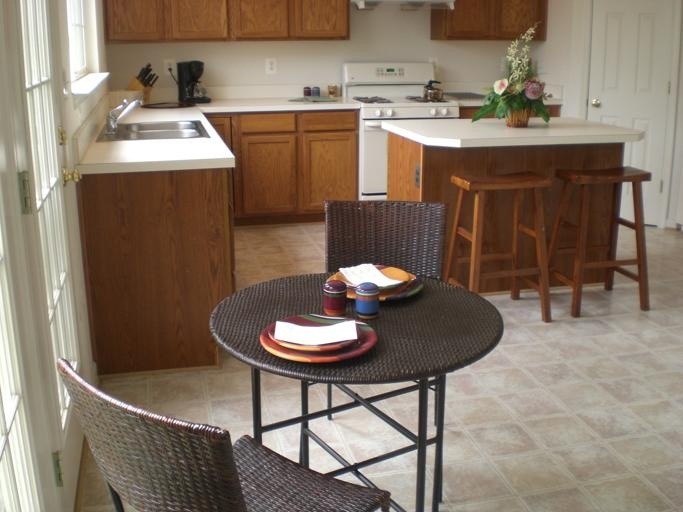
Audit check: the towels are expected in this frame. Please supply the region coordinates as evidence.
[274,320,358,345]
[338,263,403,288]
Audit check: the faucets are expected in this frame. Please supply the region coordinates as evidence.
[103,96,143,129]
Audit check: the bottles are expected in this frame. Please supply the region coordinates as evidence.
[304,86,311,96]
[312,87,320,96]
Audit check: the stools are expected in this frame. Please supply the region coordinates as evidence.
[547,165,650,316]
[441,171,553,322]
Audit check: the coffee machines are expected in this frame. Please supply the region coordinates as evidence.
[169,60,210,107]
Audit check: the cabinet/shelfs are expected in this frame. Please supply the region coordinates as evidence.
[230,0,350,39]
[106,0,230,42]
[237,112,360,216]
[429,0,547,38]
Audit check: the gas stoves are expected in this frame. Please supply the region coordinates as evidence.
[343,62,460,106]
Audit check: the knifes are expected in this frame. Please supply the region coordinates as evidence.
[139,62,159,89]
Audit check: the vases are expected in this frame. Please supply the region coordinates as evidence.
[506,107,531,128]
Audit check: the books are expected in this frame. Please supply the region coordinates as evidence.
[287,97,314,104]
[305,95,337,102]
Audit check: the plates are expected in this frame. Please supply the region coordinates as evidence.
[268,315,361,353]
[260,315,378,365]
[337,267,409,293]
[326,265,424,301]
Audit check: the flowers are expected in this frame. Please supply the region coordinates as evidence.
[472,21,553,123]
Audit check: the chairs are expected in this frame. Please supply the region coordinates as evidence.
[324,200,447,427]
[56,357,392,510]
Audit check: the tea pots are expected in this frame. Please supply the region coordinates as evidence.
[423,79,448,103]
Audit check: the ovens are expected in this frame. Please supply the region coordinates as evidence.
[359,106,459,201]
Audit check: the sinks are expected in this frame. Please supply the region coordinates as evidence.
[102,119,207,132]
[95,128,212,142]
